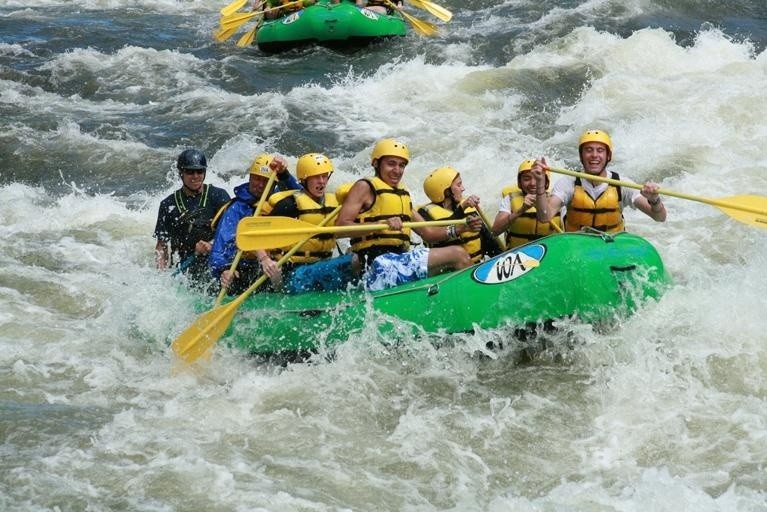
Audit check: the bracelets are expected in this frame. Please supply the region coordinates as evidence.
[535,185,545,190]
[446,224,459,239]
[647,197,661,206]
[536,192,545,196]
[508,215,514,227]
[651,204,665,213]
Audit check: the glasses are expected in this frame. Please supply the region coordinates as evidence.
[184,168,204,175]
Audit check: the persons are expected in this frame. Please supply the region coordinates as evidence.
[491,159,561,250]
[529,130,666,233]
[153,150,232,287]
[251,152,360,293]
[416,167,505,264]
[206,152,305,297]
[252,0,403,19]
[336,138,472,293]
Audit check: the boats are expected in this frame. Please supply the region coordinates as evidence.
[257,1,406,53]
[195,233,664,364]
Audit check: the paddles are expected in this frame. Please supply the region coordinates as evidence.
[235,16,262,49]
[169,202,350,368]
[534,160,767,232]
[219,0,249,18]
[387,1,434,36]
[234,213,473,255]
[416,0,452,22]
[211,0,268,44]
[219,0,304,32]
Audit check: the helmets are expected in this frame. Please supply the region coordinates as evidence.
[371,138,410,168]
[423,165,461,205]
[176,148,208,171]
[578,129,614,153]
[246,153,281,182]
[517,159,553,191]
[295,151,335,182]
